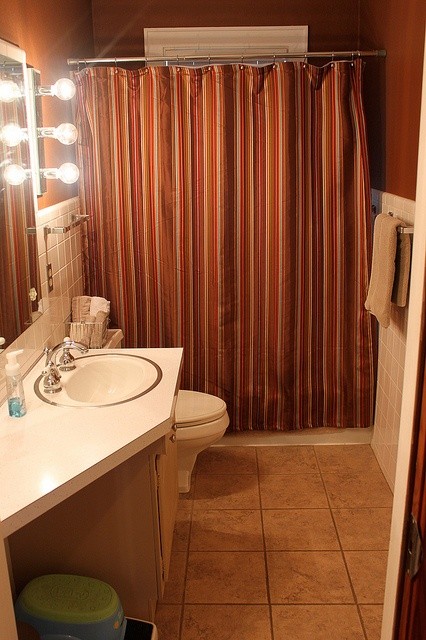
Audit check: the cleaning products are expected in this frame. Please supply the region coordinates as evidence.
[5,348,27,419]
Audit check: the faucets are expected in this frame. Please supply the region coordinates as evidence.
[45,341,88,365]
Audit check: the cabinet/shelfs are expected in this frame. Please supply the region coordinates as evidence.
[156,406,181,582]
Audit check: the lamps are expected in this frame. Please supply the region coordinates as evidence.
[36,121,78,146]
[2,78,26,102]
[35,77,79,102]
[37,160,83,185]
[6,161,31,187]
[2,121,29,147]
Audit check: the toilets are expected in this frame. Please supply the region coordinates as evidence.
[175,388,230,493]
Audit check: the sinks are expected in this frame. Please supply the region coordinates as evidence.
[35,352,163,409]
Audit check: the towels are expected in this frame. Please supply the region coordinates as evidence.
[364,213,411,329]
